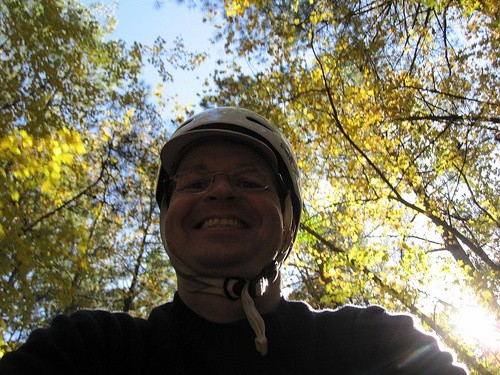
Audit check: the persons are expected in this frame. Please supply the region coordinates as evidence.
[0,106,471,375]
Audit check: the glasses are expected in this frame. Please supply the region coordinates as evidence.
[168,166,277,195]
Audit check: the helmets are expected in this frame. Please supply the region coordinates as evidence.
[155,106,302,261]
